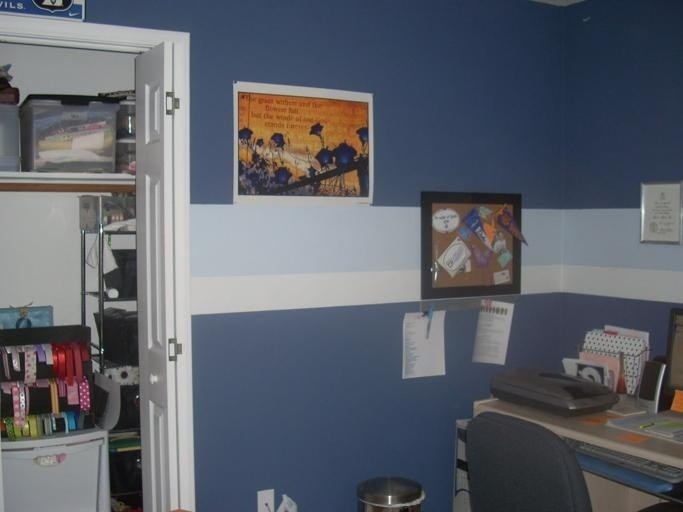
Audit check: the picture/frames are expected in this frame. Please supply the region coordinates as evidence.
[420,191,524,300]
[640,181,683,245]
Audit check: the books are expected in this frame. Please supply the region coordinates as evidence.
[606,409,683,445]
[561,352,620,393]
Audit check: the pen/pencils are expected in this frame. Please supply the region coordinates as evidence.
[427,304,434,340]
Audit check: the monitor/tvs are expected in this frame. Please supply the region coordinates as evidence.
[664,307,683,390]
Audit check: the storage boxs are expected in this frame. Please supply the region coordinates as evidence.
[0,95,135,174]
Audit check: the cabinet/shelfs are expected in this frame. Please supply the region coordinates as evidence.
[81,194,139,374]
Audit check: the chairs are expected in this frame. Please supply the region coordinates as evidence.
[464,411,592,512]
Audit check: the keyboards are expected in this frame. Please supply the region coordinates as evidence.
[575,443,683,484]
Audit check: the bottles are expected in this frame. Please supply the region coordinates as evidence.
[116,100,137,172]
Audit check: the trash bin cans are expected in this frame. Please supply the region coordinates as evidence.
[358,477,425,512]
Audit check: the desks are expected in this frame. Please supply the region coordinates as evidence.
[455,391,682,512]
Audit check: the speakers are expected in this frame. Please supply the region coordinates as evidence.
[635,360,675,415]
